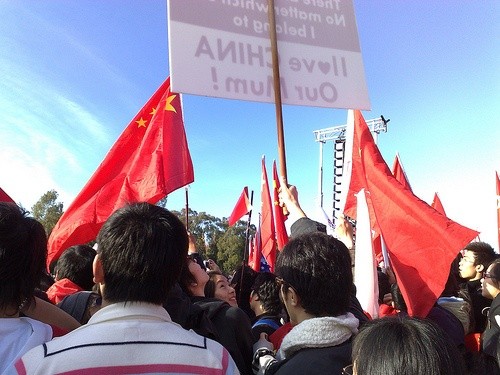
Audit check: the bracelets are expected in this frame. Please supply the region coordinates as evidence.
[19,296,30,309]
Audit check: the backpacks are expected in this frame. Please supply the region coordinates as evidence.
[253,313,292,353]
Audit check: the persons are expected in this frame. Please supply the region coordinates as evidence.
[7,203,241,375]
[167,176,500,375]
[0,201,102,375]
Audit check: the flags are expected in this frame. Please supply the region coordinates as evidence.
[373,153,412,266]
[228,187,252,227]
[343,109,481,321]
[46,77,195,273]
[494,173,500,248]
[260,158,276,273]
[249,234,261,273]
[273,161,289,252]
[431,194,447,217]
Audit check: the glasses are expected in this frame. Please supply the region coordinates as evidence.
[483,272,494,279]
[341,364,357,375]
